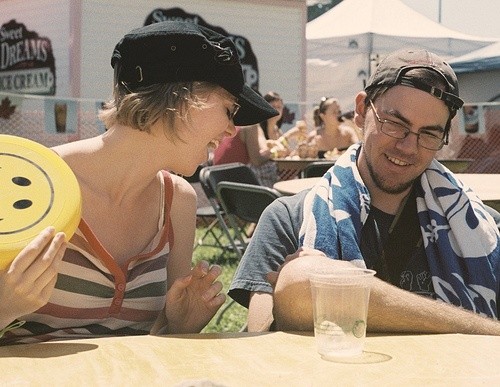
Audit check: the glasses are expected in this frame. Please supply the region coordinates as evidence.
[215,92,241,120]
[368,98,449,150]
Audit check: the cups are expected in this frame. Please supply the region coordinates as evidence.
[307,268,377,358]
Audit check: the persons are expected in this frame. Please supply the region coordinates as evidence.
[0,20,279,348]
[226,50,499,334]
[208,90,363,240]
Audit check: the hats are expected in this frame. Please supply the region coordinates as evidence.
[111,21,280,126]
[364,48,464,118]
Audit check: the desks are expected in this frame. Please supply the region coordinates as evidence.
[0,334,500,387]
[273,172,500,202]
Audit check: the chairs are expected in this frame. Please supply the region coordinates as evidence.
[170,148,336,332]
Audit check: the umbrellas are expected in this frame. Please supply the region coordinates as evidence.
[306,0,497,87]
